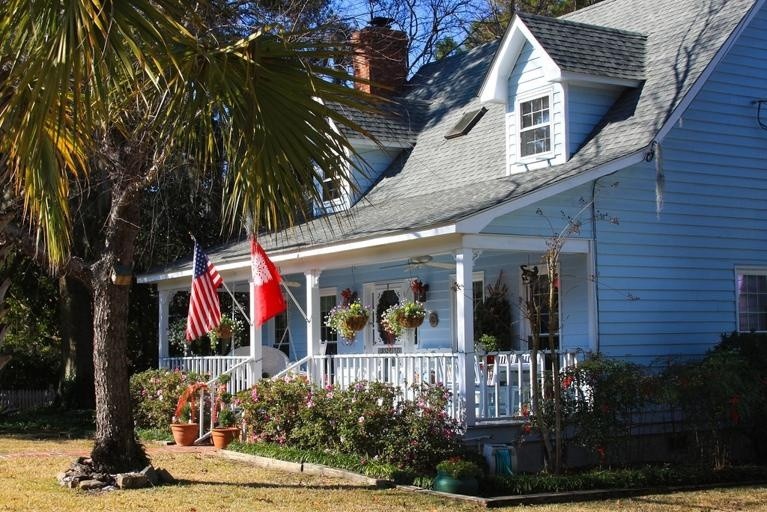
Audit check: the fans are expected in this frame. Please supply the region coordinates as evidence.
[380,254,456,272]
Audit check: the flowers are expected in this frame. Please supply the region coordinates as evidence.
[164,294,433,346]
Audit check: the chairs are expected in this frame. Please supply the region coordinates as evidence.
[405,347,544,419]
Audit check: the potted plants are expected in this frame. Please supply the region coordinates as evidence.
[475,335,498,364]
[169,371,239,449]
[432,458,483,496]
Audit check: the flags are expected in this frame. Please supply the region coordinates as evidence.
[184,237,224,343]
[248,233,288,327]
